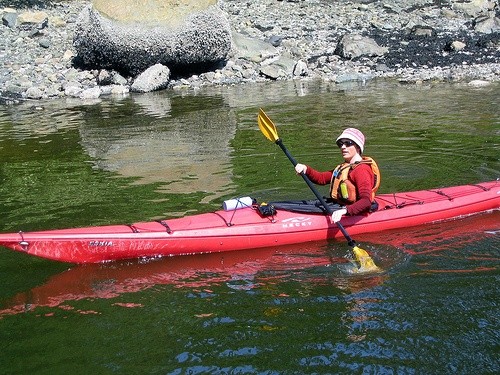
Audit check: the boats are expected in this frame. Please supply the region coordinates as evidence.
[2,209,499,320]
[1,175,499,264]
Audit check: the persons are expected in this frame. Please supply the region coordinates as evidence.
[295,128,381,226]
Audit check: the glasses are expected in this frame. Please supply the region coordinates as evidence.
[338,140,355,147]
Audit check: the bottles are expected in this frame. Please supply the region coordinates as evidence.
[223,197,256,211]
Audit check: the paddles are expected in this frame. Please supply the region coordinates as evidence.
[258,108,377,272]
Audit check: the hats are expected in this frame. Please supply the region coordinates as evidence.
[336,127,365,154]
